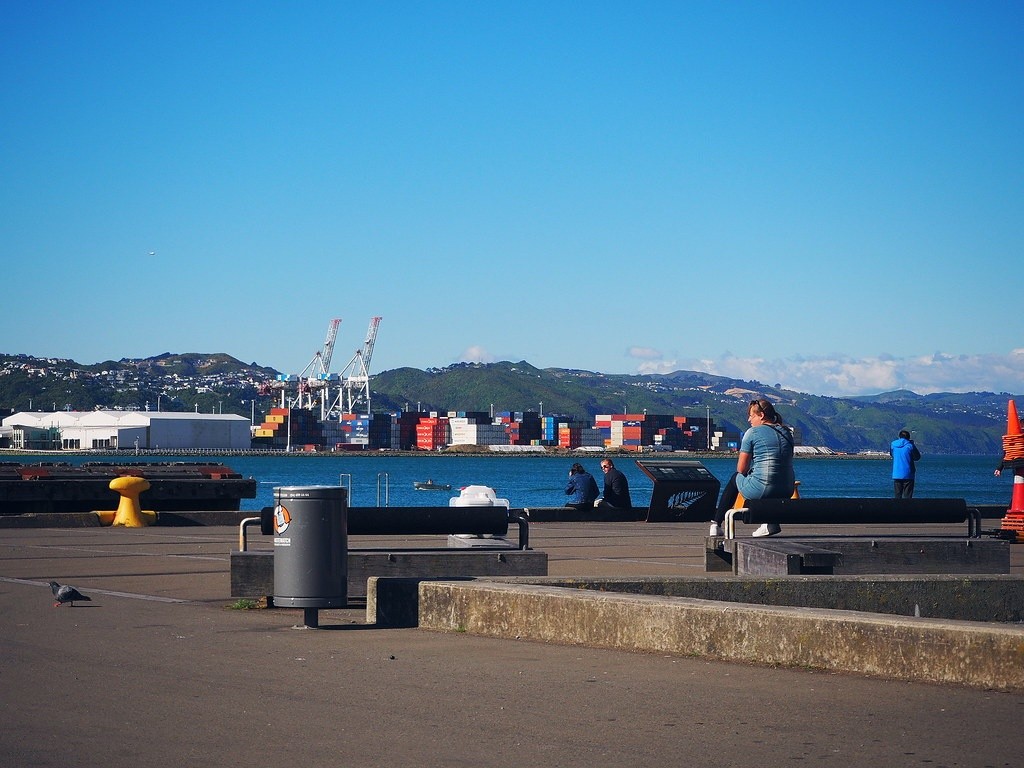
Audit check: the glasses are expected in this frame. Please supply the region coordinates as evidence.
[755,400,764,411]
[601,464,610,469]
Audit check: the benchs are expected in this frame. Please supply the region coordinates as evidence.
[238,505,529,551]
[724,496,982,540]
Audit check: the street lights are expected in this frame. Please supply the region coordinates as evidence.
[706,405,712,451]
[28,398,33,411]
[158,395,162,412]
[52,402,57,411]
[417,401,421,414]
[539,401,543,415]
[251,400,256,424]
[405,402,409,414]
[195,403,200,411]
[218,400,223,415]
[491,404,494,419]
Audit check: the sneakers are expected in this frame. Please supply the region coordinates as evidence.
[752,524,781,538]
[709,524,724,536]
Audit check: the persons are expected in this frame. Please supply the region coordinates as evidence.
[598,458,632,510]
[709,399,795,537]
[993,419,1024,518]
[564,463,600,507]
[890,430,921,499]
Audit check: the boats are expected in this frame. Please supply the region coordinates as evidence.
[0,458,258,512]
[412,480,452,491]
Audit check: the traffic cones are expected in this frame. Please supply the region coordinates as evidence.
[1002,399,1024,545]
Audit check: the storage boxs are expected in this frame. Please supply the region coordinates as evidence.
[250,408,745,452]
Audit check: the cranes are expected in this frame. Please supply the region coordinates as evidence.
[315,318,342,382]
[352,314,384,386]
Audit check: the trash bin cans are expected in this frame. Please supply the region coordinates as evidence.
[272,485,348,610]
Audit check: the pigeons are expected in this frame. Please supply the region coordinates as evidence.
[47,580,92,607]
